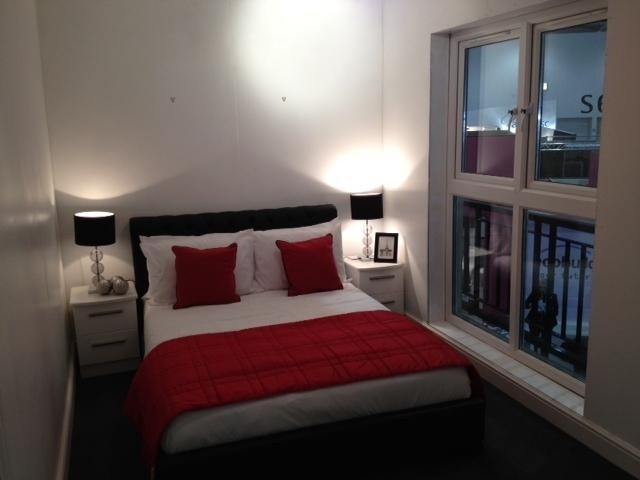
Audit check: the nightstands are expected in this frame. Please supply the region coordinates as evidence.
[344,256,405,312]
[70,281,142,379]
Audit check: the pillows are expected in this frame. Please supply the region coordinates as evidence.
[275,233,344,297]
[254,217,347,292]
[139,229,264,306]
[171,242,241,310]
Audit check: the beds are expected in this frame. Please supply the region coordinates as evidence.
[129,204,487,480]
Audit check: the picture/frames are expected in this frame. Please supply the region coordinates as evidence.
[374,232,398,264]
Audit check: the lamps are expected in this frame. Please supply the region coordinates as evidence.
[349,192,382,261]
[73,210,117,293]
